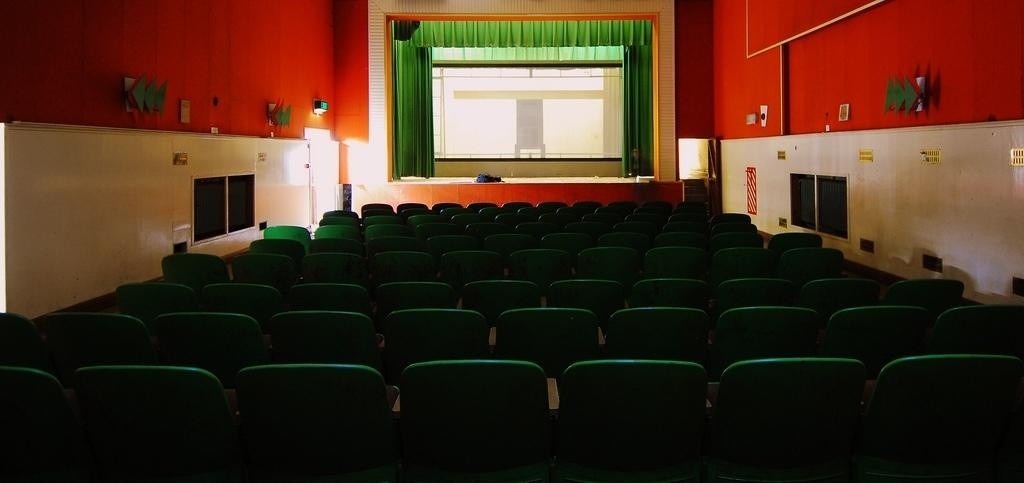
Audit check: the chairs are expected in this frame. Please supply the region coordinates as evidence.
[364,215,400,225]
[468,203,498,213]
[565,222,613,241]
[495,307,600,376]
[251,239,304,273]
[70,365,245,483]
[643,201,672,208]
[400,208,427,225]
[633,207,664,219]
[668,213,707,223]
[555,207,589,217]
[708,232,763,265]
[573,202,601,209]
[202,282,282,334]
[551,360,709,483]
[439,208,459,215]
[547,279,624,332]
[415,223,464,248]
[883,280,964,324]
[398,361,550,483]
[662,222,707,233]
[538,202,568,212]
[800,278,880,329]
[397,203,427,214]
[272,311,378,368]
[712,278,792,329]
[384,308,488,368]
[516,222,563,234]
[288,282,369,313]
[424,235,480,265]
[704,359,865,483]
[484,234,537,268]
[440,250,504,297]
[152,312,265,382]
[368,236,422,271]
[407,215,448,237]
[598,232,649,250]
[508,249,568,298]
[234,364,391,483]
[513,206,546,221]
[928,304,1024,354]
[377,281,458,334]
[309,238,362,255]
[540,233,592,251]
[672,208,705,215]
[324,211,358,216]
[0,365,97,483]
[539,213,577,225]
[582,213,617,224]
[503,202,533,212]
[767,233,822,259]
[118,283,199,336]
[782,247,844,294]
[473,205,514,223]
[607,306,708,370]
[710,247,779,278]
[624,214,666,224]
[465,223,512,235]
[711,214,750,226]
[301,253,369,283]
[576,246,640,293]
[364,224,412,238]
[495,214,538,232]
[162,254,229,293]
[432,202,463,215]
[632,278,709,309]
[44,312,159,365]
[264,227,308,255]
[462,280,545,327]
[653,232,707,248]
[821,306,929,362]
[374,251,432,282]
[362,209,392,216]
[711,306,817,382]
[712,223,755,235]
[232,253,296,288]
[859,356,1024,483]
[316,225,360,239]
[677,202,707,208]
[609,202,636,208]
[644,246,706,280]
[363,202,392,209]
[0,313,48,373]
[448,214,491,224]
[320,216,360,227]
[613,222,656,240]
[593,208,627,216]
